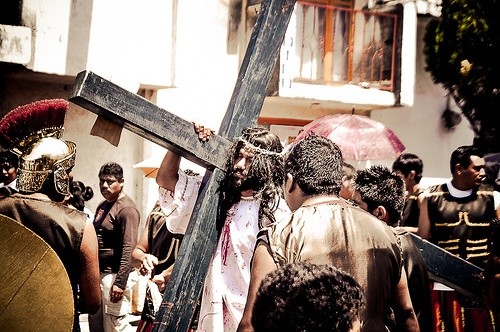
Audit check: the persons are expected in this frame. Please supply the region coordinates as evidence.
[154,126,290,332]
[0,99,102,332]
[418,146,500,332]
[130,169,202,332]
[252,262,367,332]
[392,154,425,228]
[478,154,500,191]
[353,166,431,332]
[338,163,356,202]
[235,137,420,332]
[92,162,141,332]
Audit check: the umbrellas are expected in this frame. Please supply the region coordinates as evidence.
[292,114,406,162]
